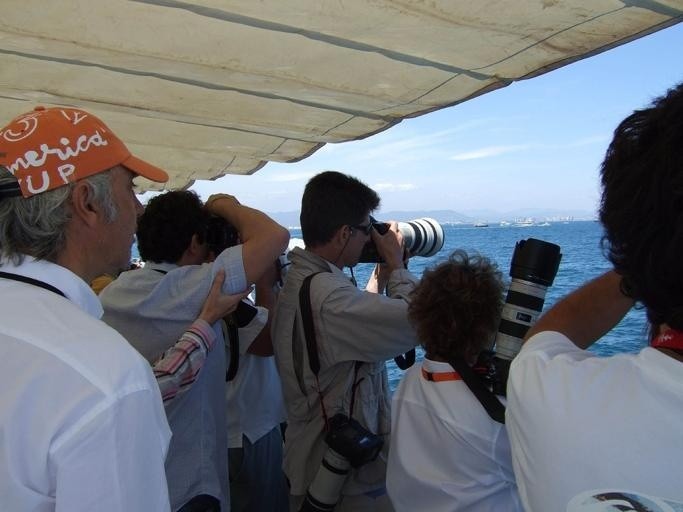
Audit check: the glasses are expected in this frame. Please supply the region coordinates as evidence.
[354,222,373,235]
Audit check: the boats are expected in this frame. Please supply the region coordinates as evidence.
[473,223,489,228]
[498,218,552,228]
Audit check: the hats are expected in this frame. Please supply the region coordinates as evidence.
[0,106,169,198]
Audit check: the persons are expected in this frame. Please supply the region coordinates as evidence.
[147,267,253,425]
[98,187,291,510]
[383,251,524,511]
[502,81,682,512]
[270,170,428,511]
[221,259,292,512]
[0,106,175,510]
